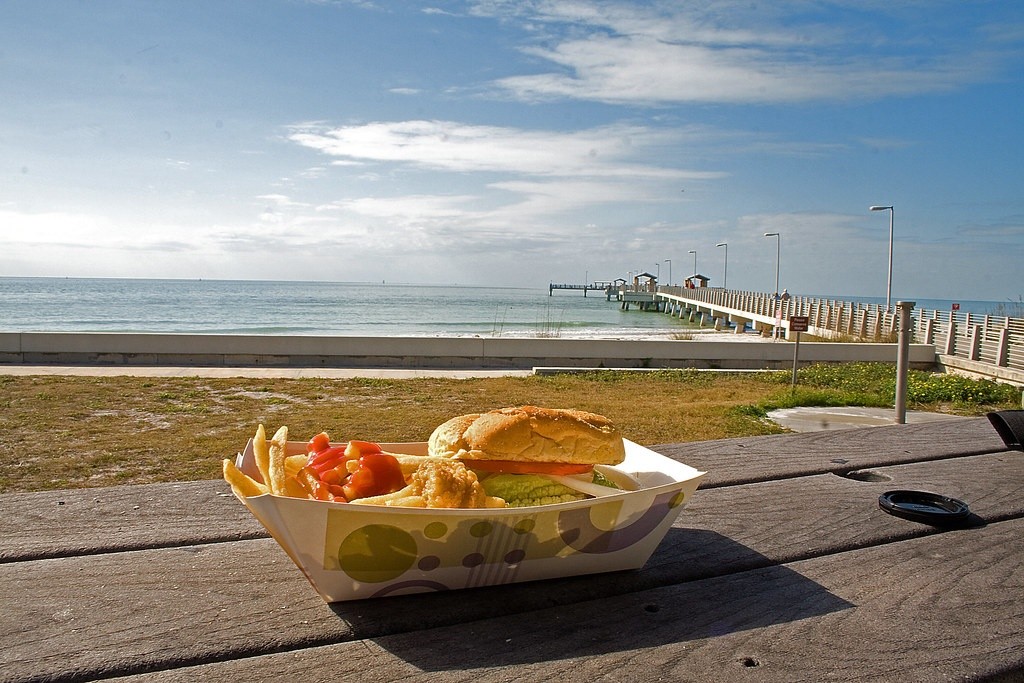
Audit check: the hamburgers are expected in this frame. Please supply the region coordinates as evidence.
[426,406,627,508]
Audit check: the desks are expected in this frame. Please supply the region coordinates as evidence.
[0,416,1023,683]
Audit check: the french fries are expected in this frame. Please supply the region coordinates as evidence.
[223,424,508,508]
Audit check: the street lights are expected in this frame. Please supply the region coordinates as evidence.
[656,263,659,283]
[689,250,697,284]
[869,205,894,310]
[765,233,779,297]
[716,243,728,289]
[665,260,671,287]
